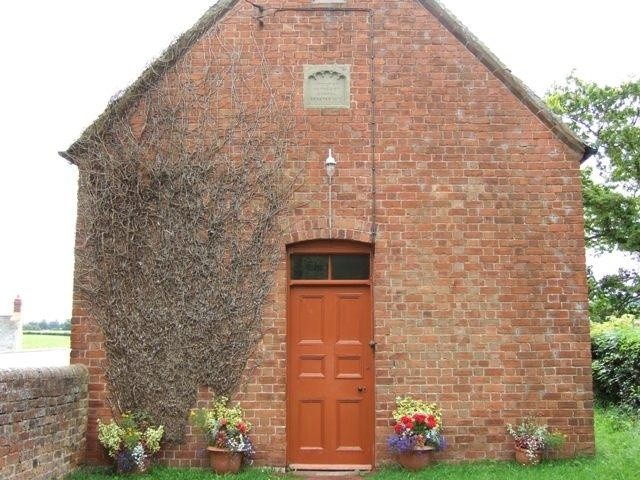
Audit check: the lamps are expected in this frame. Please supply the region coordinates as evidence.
[324,148,338,228]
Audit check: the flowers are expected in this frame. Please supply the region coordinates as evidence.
[502,409,570,463]
[97,396,257,474]
[386,396,447,457]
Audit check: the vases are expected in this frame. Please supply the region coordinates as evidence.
[513,446,544,467]
[394,445,436,470]
